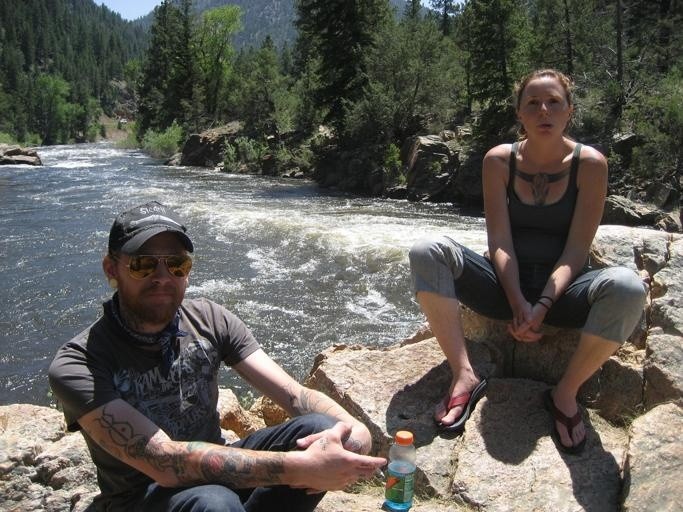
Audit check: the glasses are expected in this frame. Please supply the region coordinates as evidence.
[111,252,194,280]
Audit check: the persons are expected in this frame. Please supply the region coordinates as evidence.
[407,69,648,455]
[48,200,388,512]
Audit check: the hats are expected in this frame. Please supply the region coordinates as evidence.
[106,199,196,256]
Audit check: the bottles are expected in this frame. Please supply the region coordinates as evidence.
[383,431,417,512]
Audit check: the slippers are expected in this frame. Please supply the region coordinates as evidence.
[432,374,488,430]
[545,389,588,454]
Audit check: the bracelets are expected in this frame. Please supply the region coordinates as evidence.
[538,302,551,311]
[538,295,554,304]
